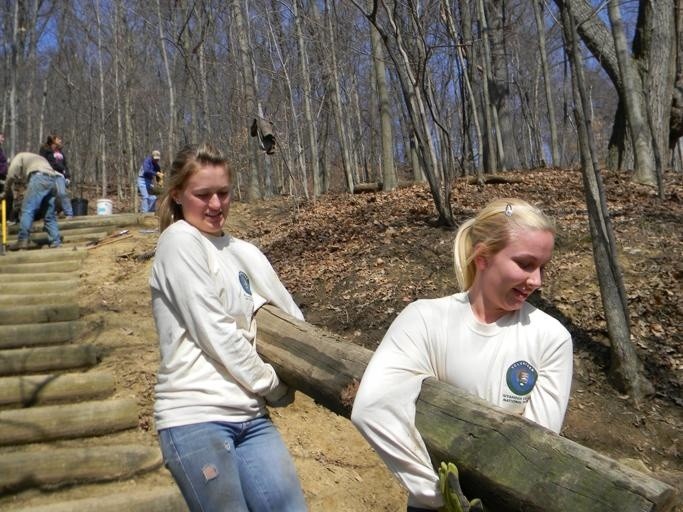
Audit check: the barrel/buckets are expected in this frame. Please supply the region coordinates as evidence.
[71,197,89,216]
[96,197,114,216]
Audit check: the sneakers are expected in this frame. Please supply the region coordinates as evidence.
[10,240,28,249]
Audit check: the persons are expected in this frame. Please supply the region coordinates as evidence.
[137,150,164,213]
[146,141,308,512]
[0,132,16,226]
[348,197,576,512]
[39,134,73,221]
[0,150,61,250]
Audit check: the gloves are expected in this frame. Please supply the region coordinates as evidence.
[437,461,484,512]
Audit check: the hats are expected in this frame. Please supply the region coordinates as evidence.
[152,151,160,160]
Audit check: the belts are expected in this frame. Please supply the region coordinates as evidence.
[28,171,55,182]
